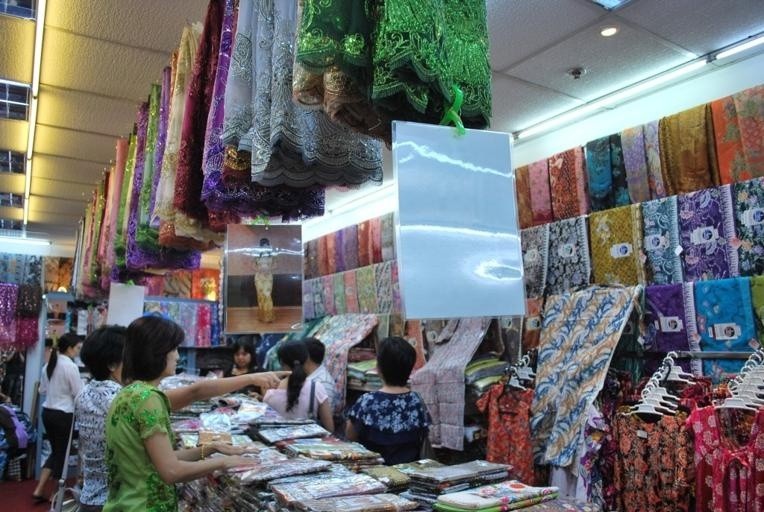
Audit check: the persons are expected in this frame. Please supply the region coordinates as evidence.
[74,323,293,512]
[252,238,279,324]
[104,315,258,512]
[31,333,82,502]
[217,338,260,395]
[263,339,336,434]
[342,336,432,466]
[302,337,337,417]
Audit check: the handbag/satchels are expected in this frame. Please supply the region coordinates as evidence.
[50,389,85,512]
[414,389,436,461]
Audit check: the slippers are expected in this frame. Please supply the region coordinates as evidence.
[31,493,47,507]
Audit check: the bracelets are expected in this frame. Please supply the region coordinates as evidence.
[201,444,204,460]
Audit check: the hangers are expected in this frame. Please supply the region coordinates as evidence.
[494,346,537,420]
[630,350,697,418]
[709,348,764,413]
